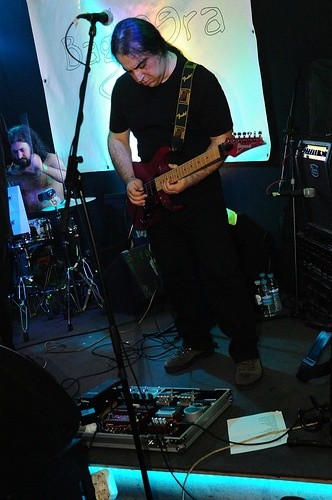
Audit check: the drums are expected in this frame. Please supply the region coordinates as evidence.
[10,217,54,247]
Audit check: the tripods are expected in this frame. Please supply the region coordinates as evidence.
[18,195,107,343]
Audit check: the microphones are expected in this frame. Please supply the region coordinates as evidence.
[271,188,317,198]
[78,10,113,26]
[287,436,332,449]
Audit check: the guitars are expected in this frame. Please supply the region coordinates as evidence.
[127,130,264,230]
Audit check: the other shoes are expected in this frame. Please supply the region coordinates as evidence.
[164,347,214,374]
[234,359,263,387]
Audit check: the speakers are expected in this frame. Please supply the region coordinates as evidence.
[295,138,332,235]
[106,244,167,315]
[0,344,83,487]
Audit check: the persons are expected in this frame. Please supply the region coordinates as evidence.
[108,18,267,392]
[6,124,82,319]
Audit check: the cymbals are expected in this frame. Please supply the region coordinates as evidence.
[40,197,96,212]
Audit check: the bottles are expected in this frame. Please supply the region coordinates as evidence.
[253,273,282,318]
